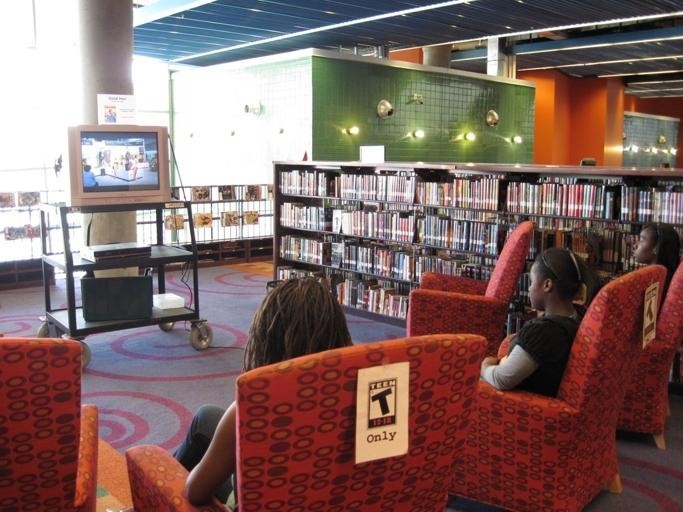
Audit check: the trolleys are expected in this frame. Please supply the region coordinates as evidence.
[35,197,214,370]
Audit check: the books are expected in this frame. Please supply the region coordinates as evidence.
[80,242,151,263]
[506,182,682,304]
[278,171,510,319]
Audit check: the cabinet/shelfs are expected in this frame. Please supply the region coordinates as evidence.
[272,160,683,328]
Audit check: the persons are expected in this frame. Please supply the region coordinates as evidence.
[84,165,98,187]
[173,276,354,510]
[113,154,138,180]
[480,248,595,397]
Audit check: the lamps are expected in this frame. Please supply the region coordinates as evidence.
[464,132,475,141]
[412,129,423,137]
[512,136,521,144]
[347,126,358,135]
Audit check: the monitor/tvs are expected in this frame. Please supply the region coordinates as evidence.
[64,124,171,207]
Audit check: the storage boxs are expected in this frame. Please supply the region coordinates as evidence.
[79,271,153,320]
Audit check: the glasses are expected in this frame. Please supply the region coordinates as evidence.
[266,279,285,294]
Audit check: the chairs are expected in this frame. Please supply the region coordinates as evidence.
[0,335,97,512]
[404,220,537,358]
[449,265,666,511]
[613,261,683,450]
[126,332,489,512]
